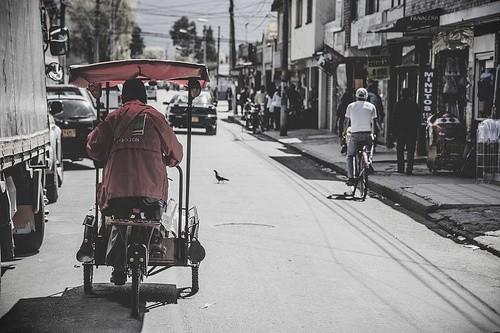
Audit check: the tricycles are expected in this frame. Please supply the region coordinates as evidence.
[240,104,268,135]
[69,59,211,317]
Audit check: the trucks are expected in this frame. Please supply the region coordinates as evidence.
[0,0,69,259]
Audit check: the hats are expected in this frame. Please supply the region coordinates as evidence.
[355,88,368,98]
[121,79,147,100]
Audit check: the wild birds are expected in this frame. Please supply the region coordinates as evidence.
[214,170,230,184]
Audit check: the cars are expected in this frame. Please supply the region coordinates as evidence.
[46,95,99,165]
[162,90,218,135]
[100,83,124,109]
[46,113,64,204]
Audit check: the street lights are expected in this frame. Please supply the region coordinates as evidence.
[197,18,208,66]
[179,28,194,53]
[244,21,251,47]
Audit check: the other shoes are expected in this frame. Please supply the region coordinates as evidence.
[346,181,354,186]
[369,163,375,175]
[396,168,413,176]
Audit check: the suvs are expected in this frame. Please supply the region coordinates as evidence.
[46,85,98,109]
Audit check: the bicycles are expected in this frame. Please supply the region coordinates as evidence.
[342,132,377,202]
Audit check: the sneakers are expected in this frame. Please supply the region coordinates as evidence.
[150,244,168,254]
[112,269,126,286]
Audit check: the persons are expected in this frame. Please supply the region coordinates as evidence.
[227,80,305,132]
[86,78,184,285]
[387,88,424,176]
[334,85,384,186]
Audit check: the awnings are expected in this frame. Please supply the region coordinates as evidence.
[366,8,447,33]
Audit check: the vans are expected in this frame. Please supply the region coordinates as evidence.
[143,83,158,102]
